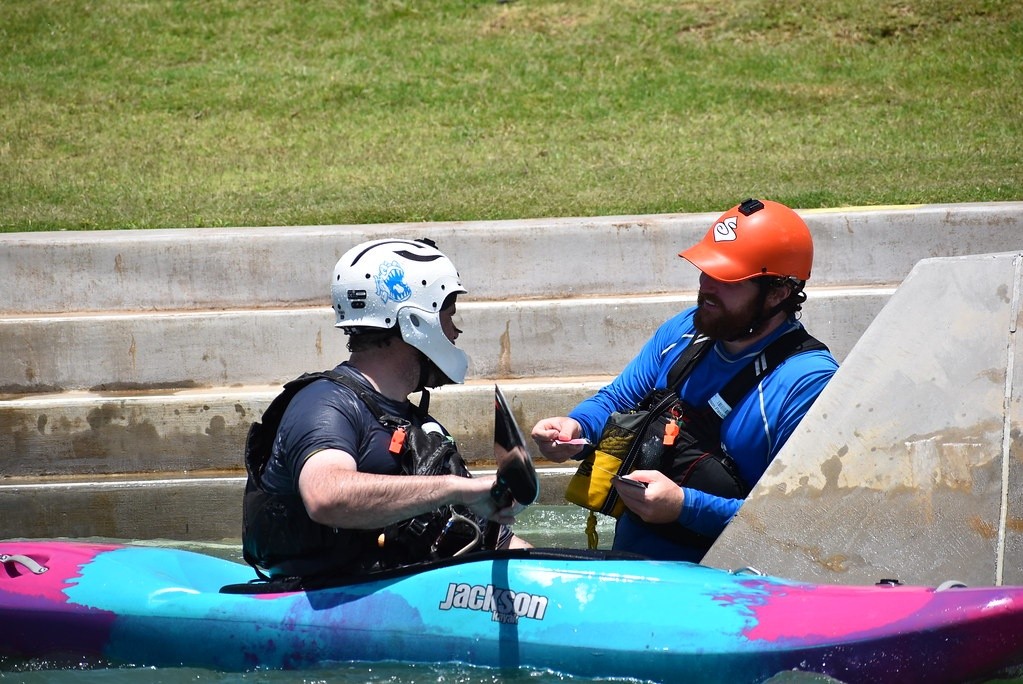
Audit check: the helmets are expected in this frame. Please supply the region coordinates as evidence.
[678,198,813,283]
[330,238,468,387]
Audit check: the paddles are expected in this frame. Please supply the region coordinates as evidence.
[484,382,540,550]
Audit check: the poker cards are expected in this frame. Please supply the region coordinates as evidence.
[618,473,646,489]
[554,437,591,446]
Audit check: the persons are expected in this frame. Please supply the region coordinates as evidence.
[531,198,841,563]
[242,238,534,586]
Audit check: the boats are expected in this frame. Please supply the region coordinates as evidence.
[0,540,1023,684]
